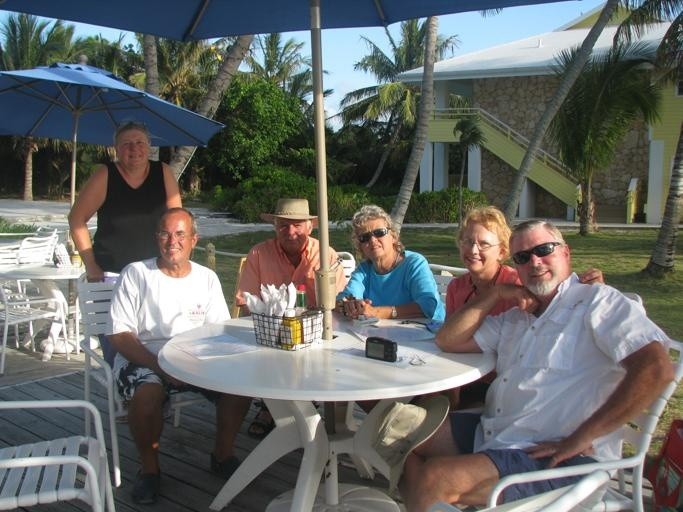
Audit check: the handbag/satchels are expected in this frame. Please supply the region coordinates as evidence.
[650,419,682,511]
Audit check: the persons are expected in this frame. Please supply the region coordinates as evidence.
[353,203,605,413]
[332,204,448,473]
[398,216,675,512]
[104,206,263,506]
[234,198,348,439]
[66,120,182,424]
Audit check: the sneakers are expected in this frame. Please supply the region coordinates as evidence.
[210,452,258,489]
[131,469,160,504]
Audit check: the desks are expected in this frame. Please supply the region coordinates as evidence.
[1,262,100,354]
[158,315,497,512]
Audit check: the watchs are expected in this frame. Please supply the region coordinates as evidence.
[390,305,397,319]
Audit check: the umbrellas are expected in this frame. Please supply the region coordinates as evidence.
[0,53,226,256]
[0,0,583,342]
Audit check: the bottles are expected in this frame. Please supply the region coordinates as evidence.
[70,251,82,268]
[295,285,305,307]
[279,310,301,347]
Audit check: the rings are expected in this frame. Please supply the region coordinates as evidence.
[549,446,555,454]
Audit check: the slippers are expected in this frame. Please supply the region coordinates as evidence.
[249,408,274,438]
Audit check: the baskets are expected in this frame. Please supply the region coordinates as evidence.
[51,254,83,268]
[251,309,324,352]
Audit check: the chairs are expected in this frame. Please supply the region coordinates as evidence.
[0,401,115,512]
[336,251,683,512]
[1,227,79,375]
[78,273,208,486]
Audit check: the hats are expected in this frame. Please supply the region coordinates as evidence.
[260,199,318,229]
[371,395,451,494]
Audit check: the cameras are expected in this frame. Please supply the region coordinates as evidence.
[366,337,397,362]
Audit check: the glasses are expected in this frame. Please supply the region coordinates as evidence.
[357,228,392,242]
[157,231,185,239]
[462,239,501,250]
[513,242,562,264]
